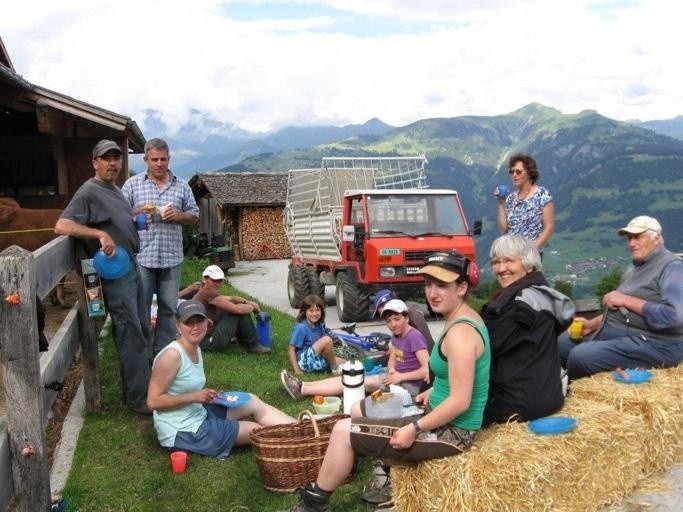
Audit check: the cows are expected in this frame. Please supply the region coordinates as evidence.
[0,198,71,308]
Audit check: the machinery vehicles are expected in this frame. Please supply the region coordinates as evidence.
[280,155,482,324]
[190,233,235,276]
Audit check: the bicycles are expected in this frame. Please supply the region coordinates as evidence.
[324,323,393,361]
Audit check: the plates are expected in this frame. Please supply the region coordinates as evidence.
[611,369,652,383]
[214,390,251,409]
[527,416,577,435]
[93,246,131,280]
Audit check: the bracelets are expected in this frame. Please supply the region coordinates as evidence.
[412,419,423,436]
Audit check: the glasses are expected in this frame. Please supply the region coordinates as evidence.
[509,170,529,175]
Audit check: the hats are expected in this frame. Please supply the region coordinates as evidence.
[203,265,225,280]
[415,250,467,283]
[618,215,662,236]
[372,289,393,320]
[93,139,124,160]
[381,299,409,313]
[177,301,207,323]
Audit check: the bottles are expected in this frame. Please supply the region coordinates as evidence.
[378,367,392,391]
[256,312,271,347]
[340,353,364,417]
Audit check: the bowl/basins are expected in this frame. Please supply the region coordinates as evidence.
[311,396,340,417]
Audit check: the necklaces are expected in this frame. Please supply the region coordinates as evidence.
[517,187,533,202]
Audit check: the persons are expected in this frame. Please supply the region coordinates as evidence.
[299,248,494,508]
[475,234,578,427]
[152,279,202,300]
[557,214,683,385]
[289,293,353,375]
[120,136,200,367]
[146,299,302,457]
[277,300,427,403]
[494,153,557,267]
[367,287,436,394]
[54,138,157,418]
[190,263,272,359]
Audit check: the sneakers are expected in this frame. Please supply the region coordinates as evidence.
[247,347,271,353]
[361,477,393,503]
[280,370,303,400]
[129,404,148,414]
[332,363,343,376]
[283,487,332,512]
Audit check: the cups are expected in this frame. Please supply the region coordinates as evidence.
[497,185,507,198]
[157,206,169,218]
[568,320,584,339]
[136,213,147,230]
[171,450,187,474]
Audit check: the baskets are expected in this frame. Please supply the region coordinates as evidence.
[248,410,351,492]
